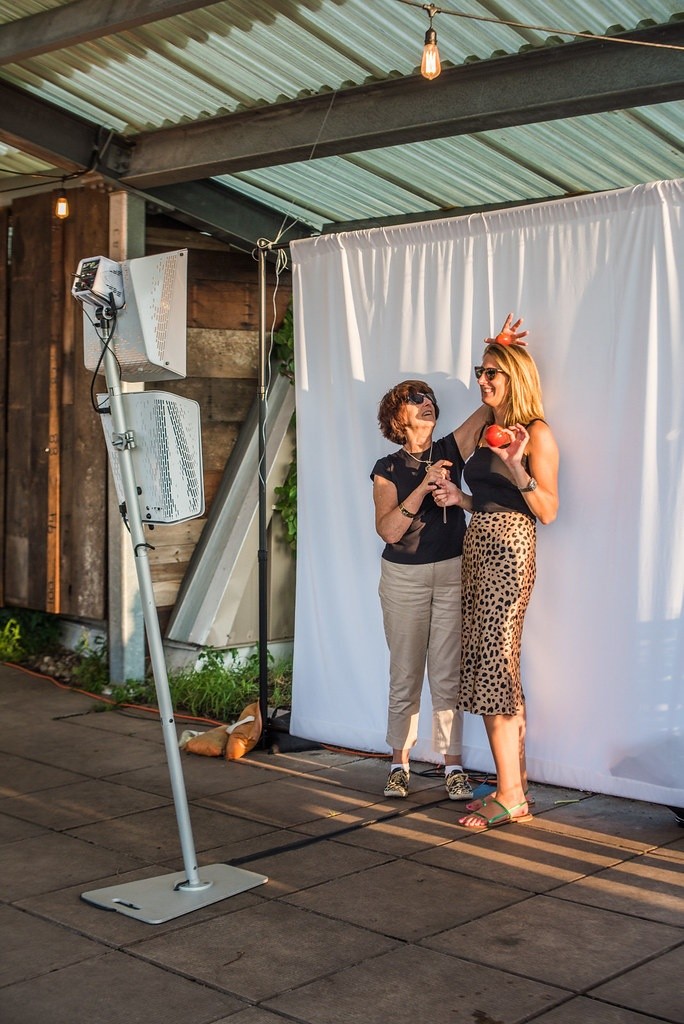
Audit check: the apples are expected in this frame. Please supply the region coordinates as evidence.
[484,424,509,448]
[495,333,512,345]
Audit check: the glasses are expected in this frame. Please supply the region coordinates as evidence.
[474,366,504,381]
[402,393,437,405]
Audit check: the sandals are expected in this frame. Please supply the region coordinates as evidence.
[456,800,533,829]
[465,790,536,813]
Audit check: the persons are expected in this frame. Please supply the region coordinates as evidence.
[428,342,560,829]
[372,311,529,801]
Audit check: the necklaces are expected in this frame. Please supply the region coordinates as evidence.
[402,440,433,472]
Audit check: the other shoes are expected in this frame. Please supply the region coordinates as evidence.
[384,767,409,797]
[446,769,474,800]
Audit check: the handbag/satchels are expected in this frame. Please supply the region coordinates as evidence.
[268,702,326,753]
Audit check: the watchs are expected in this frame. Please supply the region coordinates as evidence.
[518,477,537,492]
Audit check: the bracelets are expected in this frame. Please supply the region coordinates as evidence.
[399,503,416,518]
